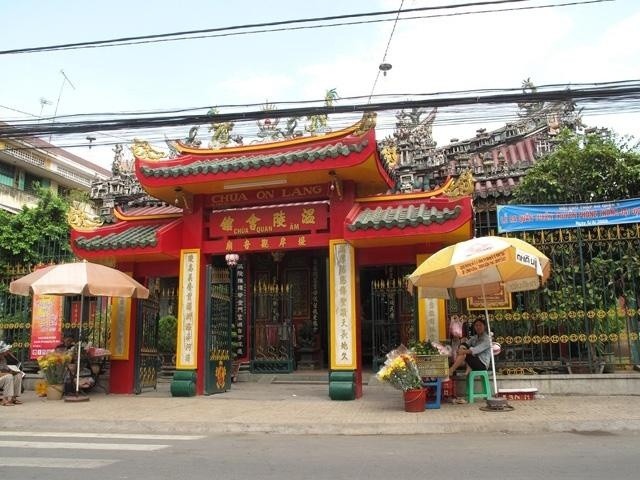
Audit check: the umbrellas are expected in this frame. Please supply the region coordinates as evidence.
[405,235,552,412]
[8,259,150,402]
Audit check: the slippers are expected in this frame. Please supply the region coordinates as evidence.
[12,400,23,404]
[0,401,14,406]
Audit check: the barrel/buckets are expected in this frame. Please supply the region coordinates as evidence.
[403,389,427,412]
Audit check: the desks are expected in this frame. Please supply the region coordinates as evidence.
[81,352,112,395]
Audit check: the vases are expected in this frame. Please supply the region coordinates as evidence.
[45,385,65,399]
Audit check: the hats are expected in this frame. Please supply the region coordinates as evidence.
[0,341,13,354]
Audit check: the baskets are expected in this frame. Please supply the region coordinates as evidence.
[415,354,449,378]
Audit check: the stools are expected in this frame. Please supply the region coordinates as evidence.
[420,378,442,409]
[466,371,492,404]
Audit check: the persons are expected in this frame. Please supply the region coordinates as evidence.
[449,318,491,377]
[0,341,23,406]
[54,335,76,350]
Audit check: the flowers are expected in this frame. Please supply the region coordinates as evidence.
[374,344,425,391]
[38,352,73,384]
[408,338,450,355]
